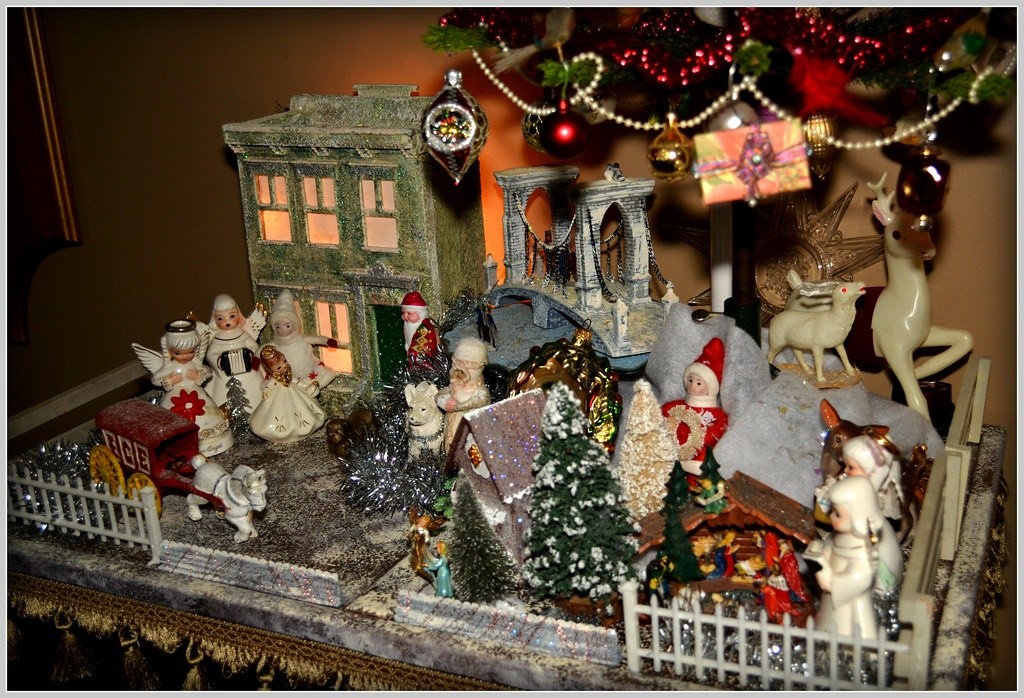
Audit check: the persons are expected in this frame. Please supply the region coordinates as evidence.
[205,294,264,416]
[816,476,877,641]
[713,532,739,577]
[401,292,441,371]
[270,289,338,390]
[250,346,327,443]
[409,515,435,562]
[842,435,903,593]
[151,319,234,439]
[423,541,454,597]
[772,538,808,603]
[660,338,728,487]
[435,338,491,457]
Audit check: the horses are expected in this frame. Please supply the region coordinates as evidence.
[186,453,268,543]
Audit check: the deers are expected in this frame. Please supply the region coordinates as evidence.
[767,281,866,382]
[816,398,928,544]
[831,170,973,461]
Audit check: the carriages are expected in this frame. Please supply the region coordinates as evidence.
[95,397,269,544]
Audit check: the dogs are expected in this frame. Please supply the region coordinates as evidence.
[405,379,444,460]
